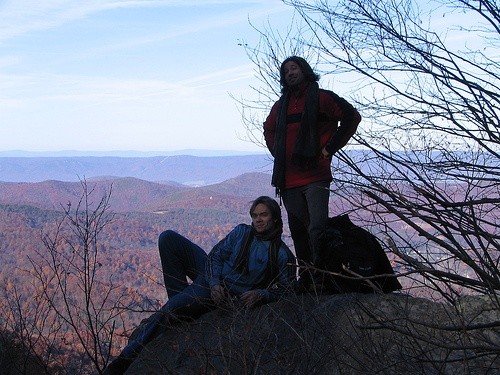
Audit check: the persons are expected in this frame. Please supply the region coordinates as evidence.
[103,196,299,375]
[262,56,362,292]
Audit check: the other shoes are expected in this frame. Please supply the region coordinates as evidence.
[107,350,133,375]
[295,276,312,296]
